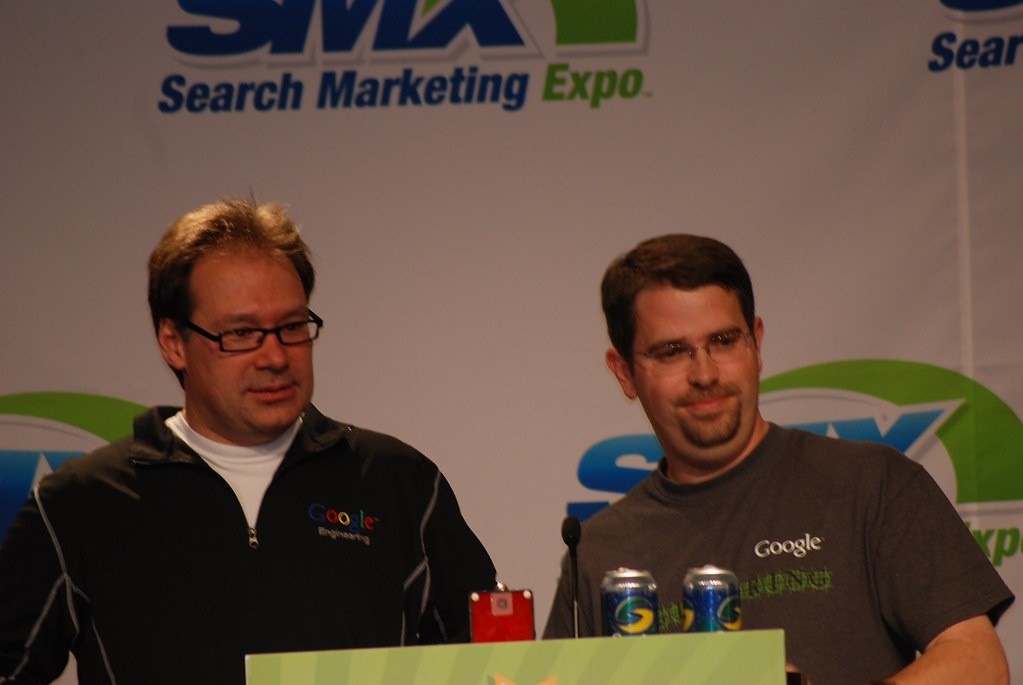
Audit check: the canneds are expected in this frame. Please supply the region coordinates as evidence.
[681,564,743,632]
[599,567,658,635]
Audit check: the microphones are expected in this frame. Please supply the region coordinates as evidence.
[562,516,582,638]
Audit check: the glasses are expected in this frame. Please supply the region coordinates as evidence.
[176,307,324,352]
[629,325,751,374]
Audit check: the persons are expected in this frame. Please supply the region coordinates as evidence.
[541,232,1015,684]
[0,194,503,684]
